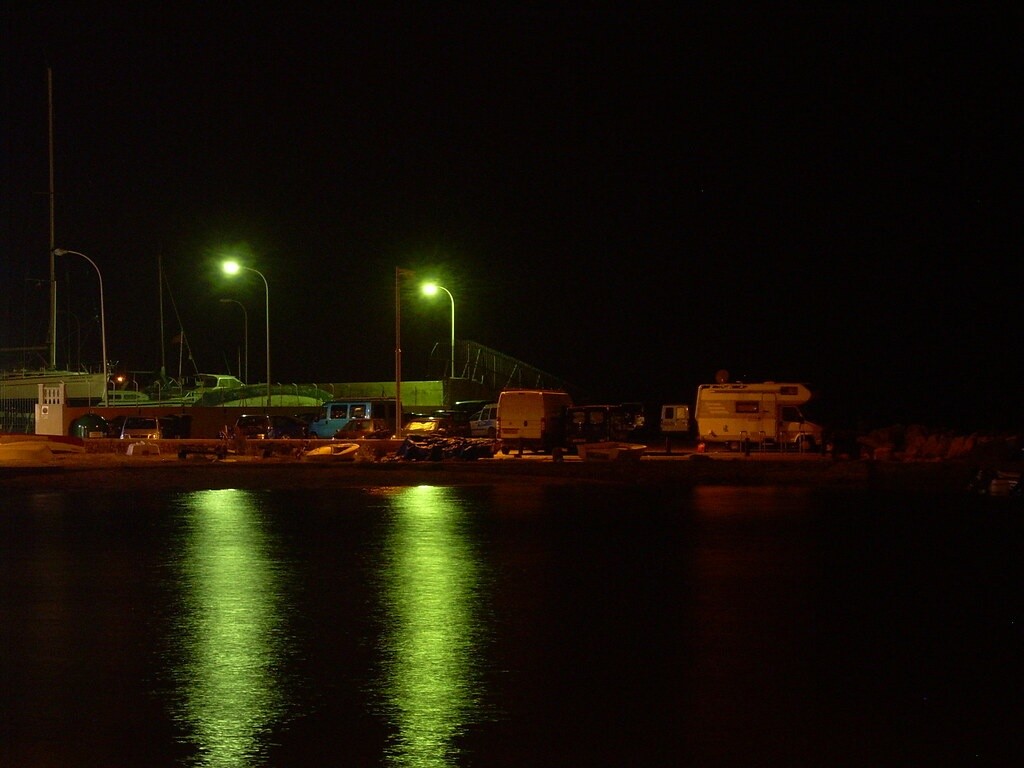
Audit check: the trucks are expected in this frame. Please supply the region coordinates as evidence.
[661,404,693,432]
[695,381,826,452]
[496,387,571,454]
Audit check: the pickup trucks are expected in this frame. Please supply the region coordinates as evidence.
[459,405,496,438]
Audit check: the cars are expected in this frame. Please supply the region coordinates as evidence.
[118,416,165,440]
[391,408,468,438]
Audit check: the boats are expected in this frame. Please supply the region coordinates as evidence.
[572,441,648,462]
[303,442,359,463]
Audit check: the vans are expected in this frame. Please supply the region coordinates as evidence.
[309,399,404,439]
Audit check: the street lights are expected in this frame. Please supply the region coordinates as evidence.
[220,298,248,387]
[54,249,109,408]
[224,260,272,405]
[423,286,455,377]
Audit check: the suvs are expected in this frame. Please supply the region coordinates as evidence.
[330,418,389,439]
[235,413,309,440]
